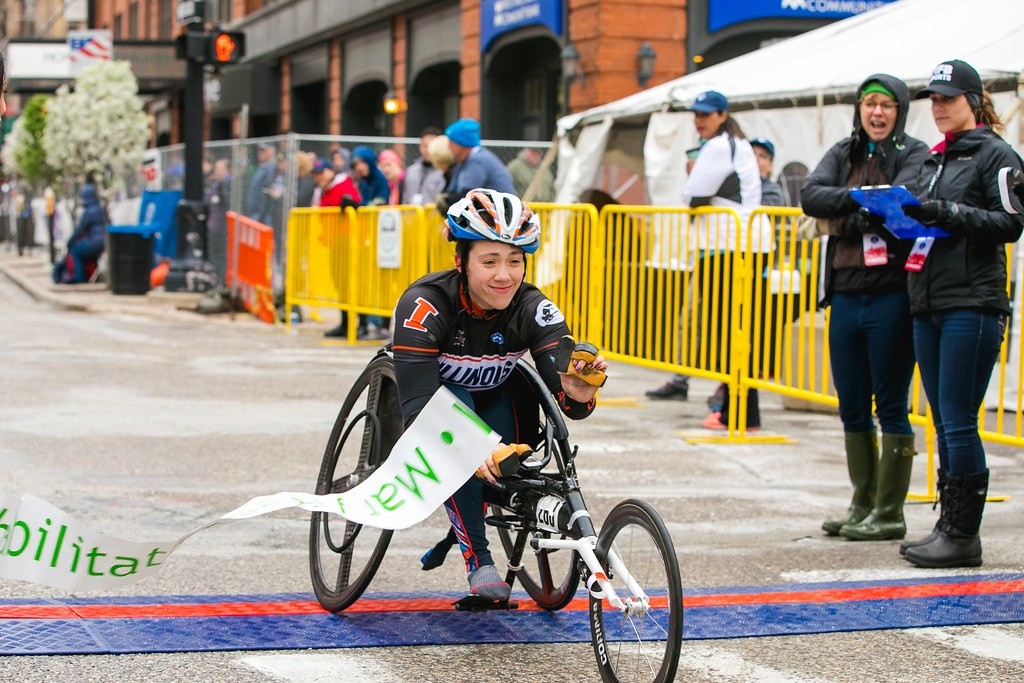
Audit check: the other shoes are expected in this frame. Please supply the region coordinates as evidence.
[705,389,723,411]
[323,322,347,336]
[703,412,760,432]
[643,381,687,400]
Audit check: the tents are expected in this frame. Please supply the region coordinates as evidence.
[546,0,1023,418]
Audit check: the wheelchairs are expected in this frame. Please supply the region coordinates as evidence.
[306,339,685,683]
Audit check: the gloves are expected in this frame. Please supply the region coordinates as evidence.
[902,201,938,226]
[857,207,884,233]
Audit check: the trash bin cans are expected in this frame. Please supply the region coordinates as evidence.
[107,225,155,295]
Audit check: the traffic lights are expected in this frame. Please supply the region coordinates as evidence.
[209,29,245,66]
[175,32,206,61]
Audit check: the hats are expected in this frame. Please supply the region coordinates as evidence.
[914,60,982,99]
[444,118,480,146]
[311,159,331,172]
[688,90,728,112]
[380,150,403,167]
[749,137,774,155]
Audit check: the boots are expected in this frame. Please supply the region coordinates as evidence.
[839,434,915,540]
[899,461,946,555]
[821,428,879,533]
[905,468,989,567]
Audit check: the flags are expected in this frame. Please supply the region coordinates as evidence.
[65,29,112,63]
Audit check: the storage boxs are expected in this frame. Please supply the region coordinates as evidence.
[782,305,933,421]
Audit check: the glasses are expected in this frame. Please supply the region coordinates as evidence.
[861,100,898,113]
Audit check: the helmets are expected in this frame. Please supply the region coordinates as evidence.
[442,189,541,254]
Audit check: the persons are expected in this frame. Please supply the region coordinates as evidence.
[858,61,1023,570]
[363,185,606,605]
[645,91,791,433]
[1,123,564,344]
[800,73,937,539]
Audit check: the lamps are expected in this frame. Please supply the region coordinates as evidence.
[561,39,589,90]
[634,38,657,86]
[381,86,406,117]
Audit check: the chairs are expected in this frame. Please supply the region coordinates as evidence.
[83,250,110,285]
